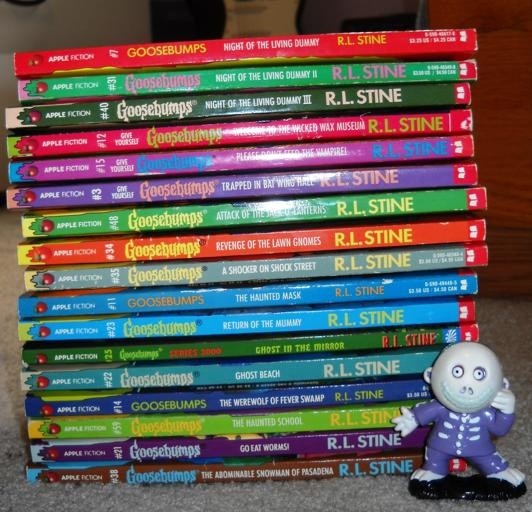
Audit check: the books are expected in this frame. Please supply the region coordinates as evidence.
[2,25,483,481]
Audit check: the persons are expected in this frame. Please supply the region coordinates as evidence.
[390,340,527,502]
